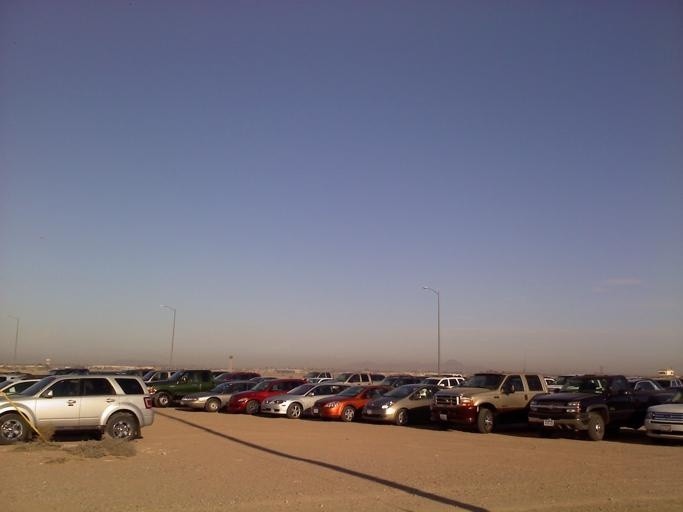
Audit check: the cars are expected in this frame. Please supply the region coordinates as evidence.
[0,370,155,397]
[644,390,682,444]
[540,374,683,394]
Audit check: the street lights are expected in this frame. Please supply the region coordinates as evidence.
[7,314,19,370]
[422,286,442,377]
[160,305,177,367]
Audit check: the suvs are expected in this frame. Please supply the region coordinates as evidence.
[0,375,154,444]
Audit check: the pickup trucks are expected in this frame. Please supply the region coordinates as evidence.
[430,371,547,434]
[527,373,677,441]
